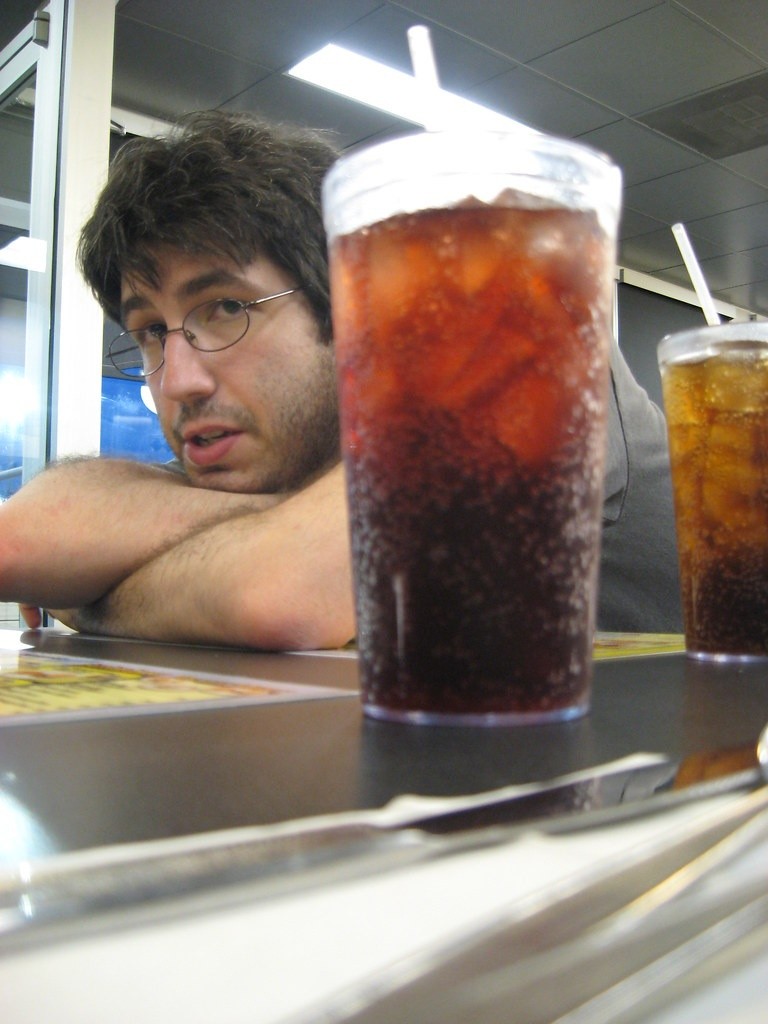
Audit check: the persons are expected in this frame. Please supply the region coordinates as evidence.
[0,112,688,646]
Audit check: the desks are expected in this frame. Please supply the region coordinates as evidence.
[0,622,768,1024]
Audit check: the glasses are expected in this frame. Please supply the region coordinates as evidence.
[104,284,305,377]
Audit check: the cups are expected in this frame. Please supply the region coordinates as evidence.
[655,322,768,665]
[319,131,624,727]
